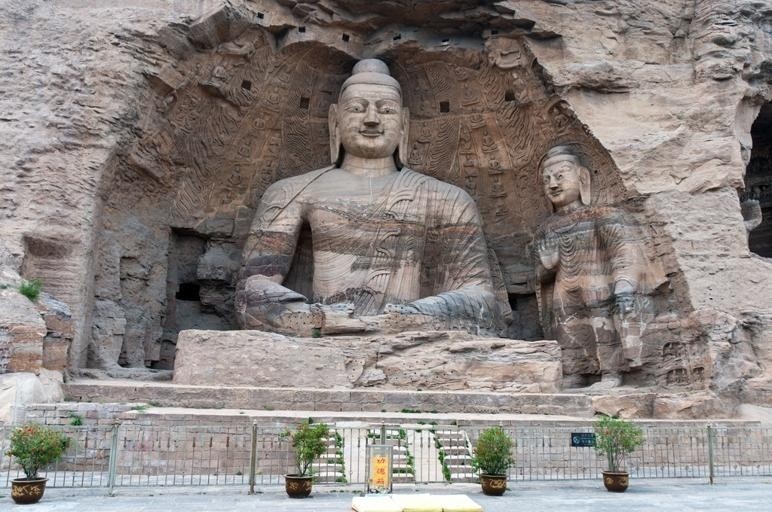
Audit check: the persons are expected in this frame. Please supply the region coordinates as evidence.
[234,59,498,335]
[529,145,646,375]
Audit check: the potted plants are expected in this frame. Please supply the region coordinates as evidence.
[470,426,517,497]
[591,415,648,493]
[4,424,71,505]
[277,424,330,500]
